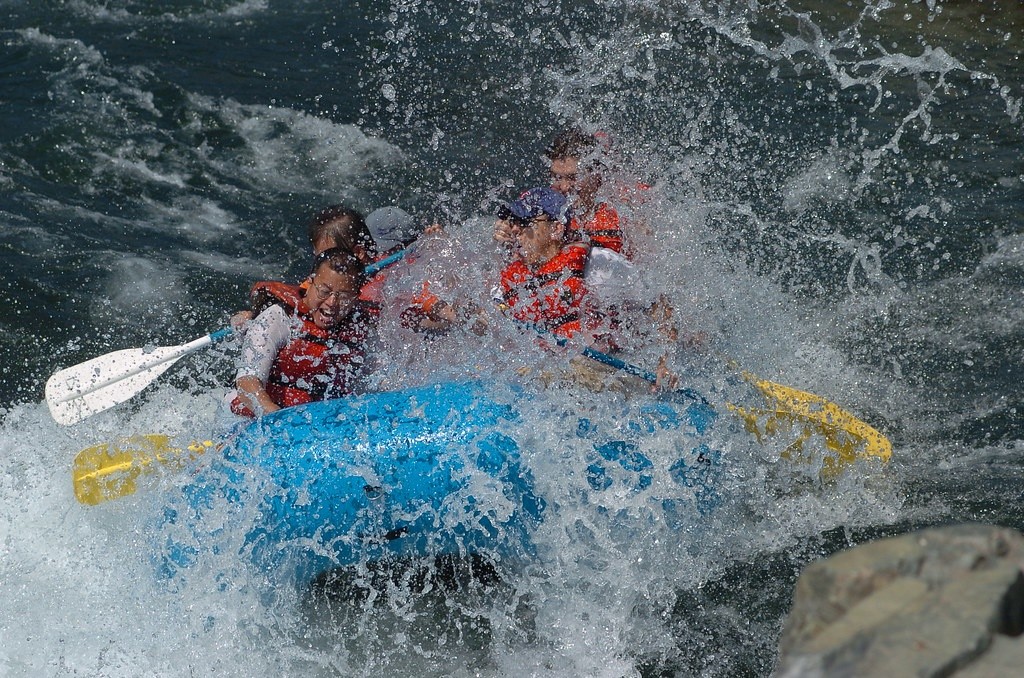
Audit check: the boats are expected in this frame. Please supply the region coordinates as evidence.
[143,371,736,605]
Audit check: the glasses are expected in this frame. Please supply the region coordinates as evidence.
[509,213,557,228]
[311,277,351,300]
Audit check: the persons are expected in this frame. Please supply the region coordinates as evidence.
[466,134,681,398]
[235,127,451,418]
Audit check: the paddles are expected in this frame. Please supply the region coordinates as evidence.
[46,235,424,429]
[70,432,227,508]
[477,301,891,483]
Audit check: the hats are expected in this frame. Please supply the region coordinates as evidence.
[365,206,417,252]
[504,187,571,226]
